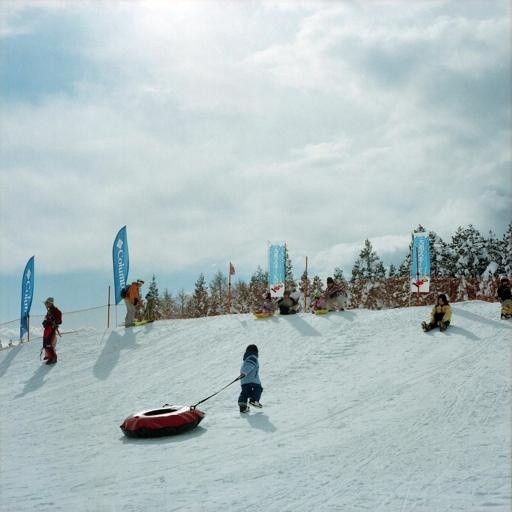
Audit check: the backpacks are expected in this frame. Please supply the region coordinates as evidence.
[121,285,131,298]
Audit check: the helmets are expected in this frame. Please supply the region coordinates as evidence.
[137,279,144,284]
[500,276,508,282]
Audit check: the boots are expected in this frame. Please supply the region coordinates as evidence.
[437,321,447,332]
[421,321,430,332]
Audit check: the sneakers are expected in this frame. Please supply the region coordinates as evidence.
[249,399,262,408]
[125,323,135,326]
[44,357,57,364]
[240,405,250,412]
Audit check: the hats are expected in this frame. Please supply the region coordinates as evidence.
[45,297,53,303]
[327,278,333,283]
[246,345,258,353]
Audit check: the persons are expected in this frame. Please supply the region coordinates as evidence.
[255,282,300,315]
[498,278,512,319]
[41,296,62,365]
[310,276,347,313]
[123,280,144,326]
[421,294,451,332]
[237,344,263,412]
